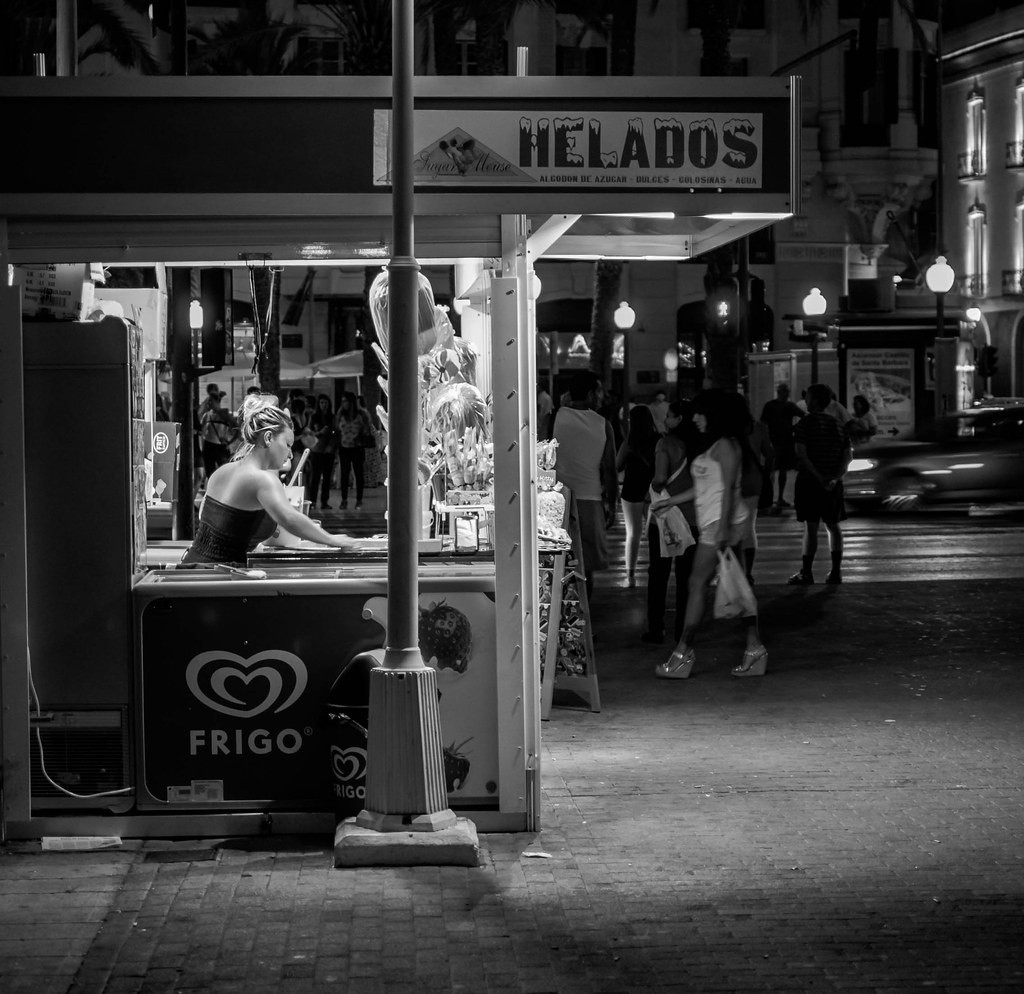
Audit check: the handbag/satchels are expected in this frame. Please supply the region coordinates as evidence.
[330,416,341,448]
[222,443,230,459]
[354,425,376,448]
[712,546,757,618]
[648,484,696,557]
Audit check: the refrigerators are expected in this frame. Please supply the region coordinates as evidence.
[23,315,495,813]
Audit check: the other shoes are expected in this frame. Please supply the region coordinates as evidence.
[825,571,842,584]
[612,578,635,589]
[777,500,790,508]
[321,504,332,509]
[309,502,316,510]
[789,571,813,583]
[758,501,777,514]
[355,500,362,509]
[640,632,663,644]
[340,499,347,509]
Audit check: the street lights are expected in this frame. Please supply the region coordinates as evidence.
[924,255,955,411]
[611,302,637,439]
[801,288,828,385]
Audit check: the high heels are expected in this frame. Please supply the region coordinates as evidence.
[656,648,695,678]
[732,645,768,676]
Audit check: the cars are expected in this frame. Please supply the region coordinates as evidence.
[842,405,1024,524]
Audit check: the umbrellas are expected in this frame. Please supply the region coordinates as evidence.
[201,350,387,411]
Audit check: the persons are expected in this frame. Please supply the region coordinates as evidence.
[157,383,381,568]
[537,369,879,678]
